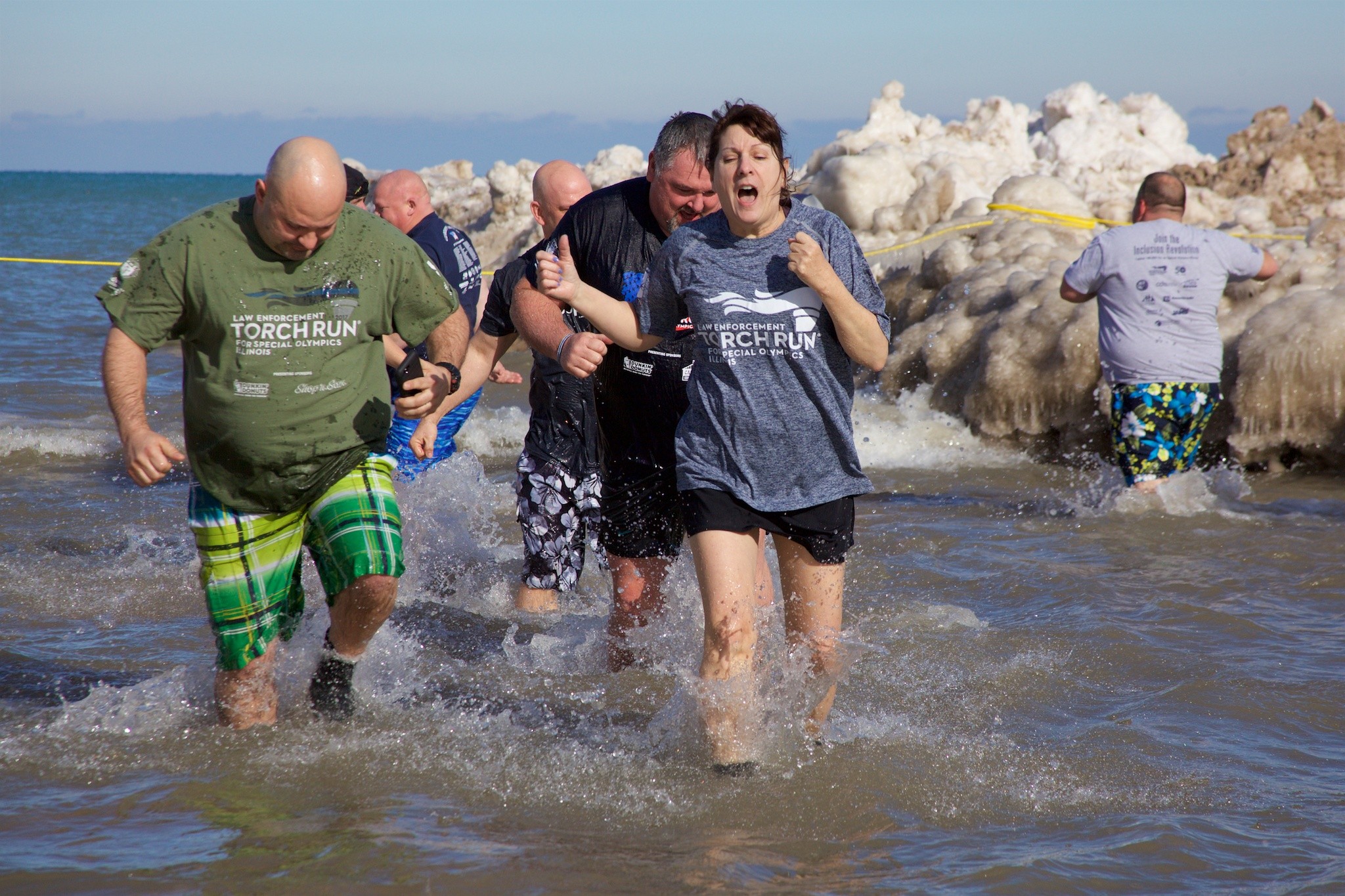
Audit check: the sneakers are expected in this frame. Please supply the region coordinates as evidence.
[307,658,357,725]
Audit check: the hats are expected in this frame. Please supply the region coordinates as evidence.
[342,162,369,202]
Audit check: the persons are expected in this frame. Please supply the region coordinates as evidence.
[408,161,607,604]
[341,163,370,210]
[536,110,721,668]
[364,170,484,481]
[1062,170,1282,492]
[529,103,892,770]
[92,135,470,727]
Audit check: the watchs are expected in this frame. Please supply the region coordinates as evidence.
[434,361,462,395]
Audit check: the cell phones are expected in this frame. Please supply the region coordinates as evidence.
[398,348,428,409]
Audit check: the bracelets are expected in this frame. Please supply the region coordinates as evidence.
[556,334,575,365]
